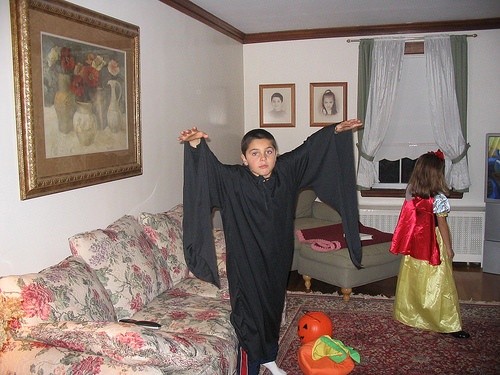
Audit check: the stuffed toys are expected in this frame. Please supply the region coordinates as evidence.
[298,335,360,375]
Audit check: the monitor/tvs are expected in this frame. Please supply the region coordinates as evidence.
[484,133,500,203]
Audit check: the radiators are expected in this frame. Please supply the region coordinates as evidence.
[359,209,485,265]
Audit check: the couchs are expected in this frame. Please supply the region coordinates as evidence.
[0,204,286,375]
[291,190,342,270]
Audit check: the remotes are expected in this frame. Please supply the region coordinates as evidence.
[134,321,161,327]
[119,319,137,324]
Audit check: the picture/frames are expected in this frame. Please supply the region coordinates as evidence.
[259,84,295,128]
[8,0,143,200]
[310,82,347,127]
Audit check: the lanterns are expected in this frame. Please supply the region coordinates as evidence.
[298,310,333,345]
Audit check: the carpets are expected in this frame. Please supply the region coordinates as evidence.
[258,290,500,375]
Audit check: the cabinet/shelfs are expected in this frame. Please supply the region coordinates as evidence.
[482,203,500,275]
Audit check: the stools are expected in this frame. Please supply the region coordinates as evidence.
[298,241,402,304]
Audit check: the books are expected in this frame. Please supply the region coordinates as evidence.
[343,233,373,240]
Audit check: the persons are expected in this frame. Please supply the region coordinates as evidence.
[177,119,364,375]
[389,150,469,338]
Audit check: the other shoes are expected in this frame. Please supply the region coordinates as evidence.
[441,331,470,338]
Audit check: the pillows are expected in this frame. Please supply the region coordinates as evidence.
[27,321,208,367]
